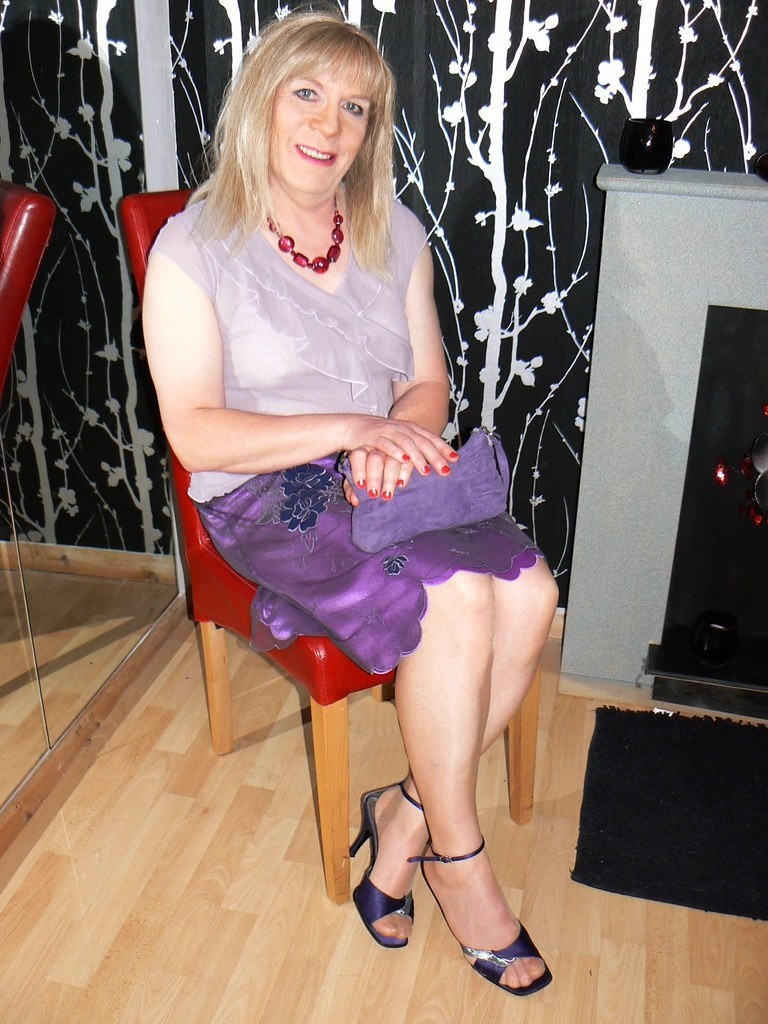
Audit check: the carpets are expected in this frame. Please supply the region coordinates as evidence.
[570,704,768,921]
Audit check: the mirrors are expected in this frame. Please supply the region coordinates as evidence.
[0,0,177,809]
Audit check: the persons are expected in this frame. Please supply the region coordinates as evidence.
[142,0,560,997]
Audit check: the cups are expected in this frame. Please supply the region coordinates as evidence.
[690,609,738,668]
[620,118,673,175]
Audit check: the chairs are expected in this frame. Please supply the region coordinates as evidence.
[119,188,539,906]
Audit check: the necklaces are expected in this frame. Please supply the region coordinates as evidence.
[266,194,344,275]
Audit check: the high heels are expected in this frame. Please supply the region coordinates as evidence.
[348,780,425,948]
[406,834,553,996]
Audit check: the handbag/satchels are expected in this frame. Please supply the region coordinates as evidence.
[336,426,508,554]
